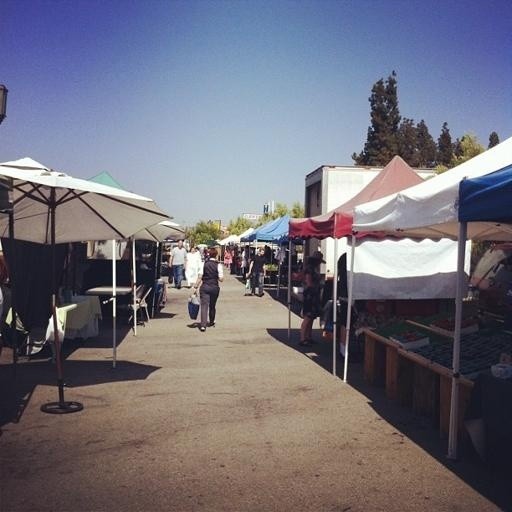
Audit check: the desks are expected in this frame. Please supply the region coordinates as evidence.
[56,295,104,341]
[86,285,133,329]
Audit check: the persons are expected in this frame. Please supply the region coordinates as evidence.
[298,250,328,347]
[0,237,11,283]
[194,246,202,269]
[224,242,279,281]
[185,247,202,289]
[245,248,267,297]
[202,246,207,259]
[167,240,188,290]
[193,248,224,331]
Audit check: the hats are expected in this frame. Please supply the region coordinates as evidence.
[306,250,327,264]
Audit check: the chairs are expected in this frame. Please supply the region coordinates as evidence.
[127,283,153,325]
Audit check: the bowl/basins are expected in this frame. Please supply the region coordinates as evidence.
[490,363,512,380]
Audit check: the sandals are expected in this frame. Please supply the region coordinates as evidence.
[298,337,318,348]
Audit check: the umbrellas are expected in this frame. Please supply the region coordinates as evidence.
[119,220,184,338]
[0,156,172,403]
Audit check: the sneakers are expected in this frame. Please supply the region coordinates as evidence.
[200,322,216,332]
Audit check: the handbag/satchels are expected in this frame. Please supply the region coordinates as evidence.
[187,298,199,320]
[168,271,173,284]
